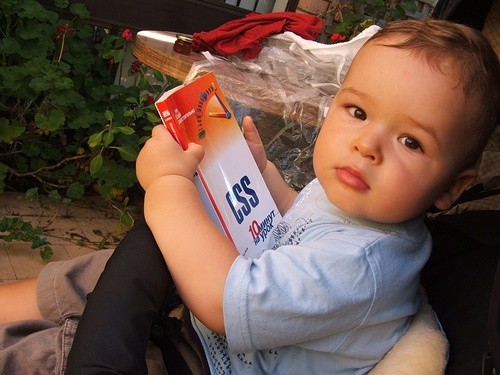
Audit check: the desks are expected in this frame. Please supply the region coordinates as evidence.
[133,31,330,151]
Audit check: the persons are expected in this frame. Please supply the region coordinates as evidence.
[1,22,499,375]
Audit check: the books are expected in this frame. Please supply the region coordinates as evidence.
[155,71,282,265]
